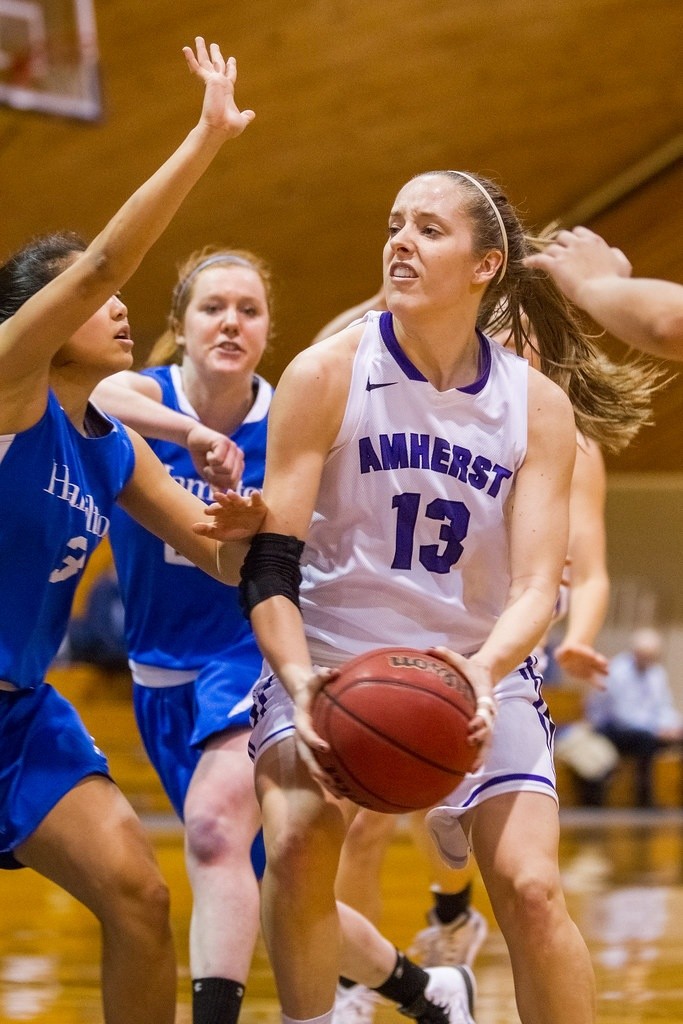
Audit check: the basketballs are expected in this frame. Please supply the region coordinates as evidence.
[308,645,483,817]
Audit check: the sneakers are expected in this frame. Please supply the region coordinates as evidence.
[329,983,377,1024]
[421,908,487,968]
[396,965,477,1024]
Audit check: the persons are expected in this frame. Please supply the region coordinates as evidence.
[0,32,683,1021]
[239,169,601,1022]
